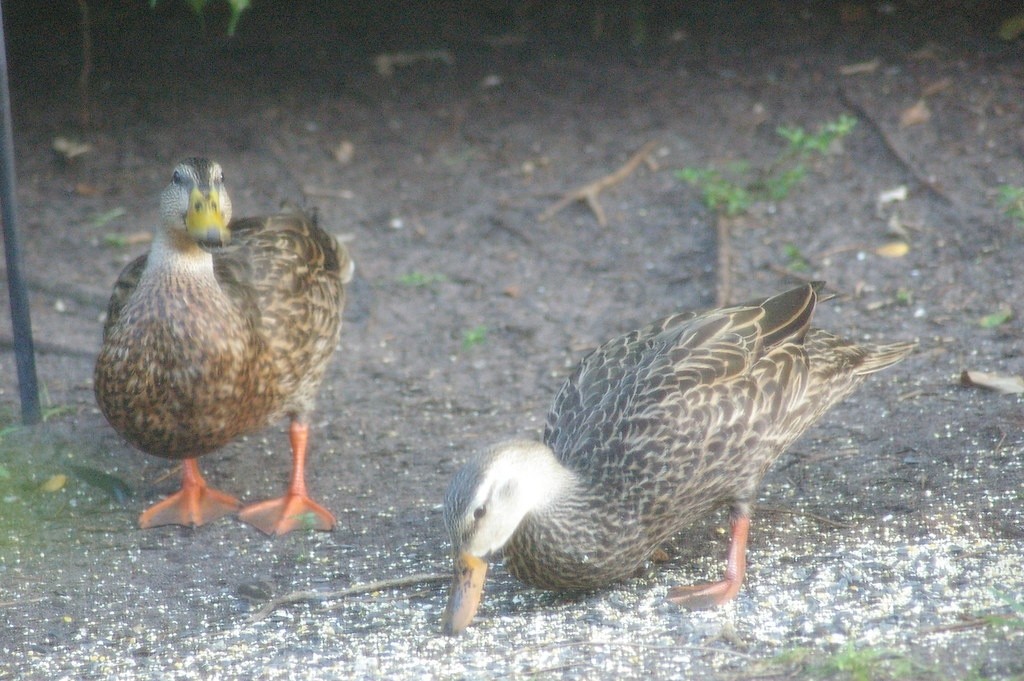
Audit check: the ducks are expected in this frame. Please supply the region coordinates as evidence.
[96,151,354,539]
[445,283,919,634]
